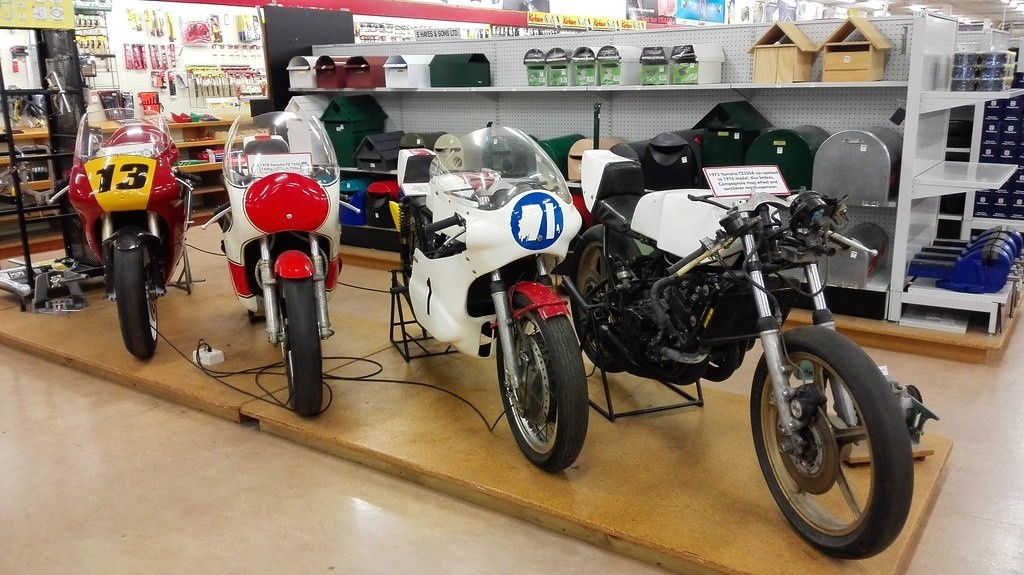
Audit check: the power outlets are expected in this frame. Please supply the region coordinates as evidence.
[192,347,226,367]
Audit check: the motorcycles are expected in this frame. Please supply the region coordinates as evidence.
[47,106,200,362]
[367,124,589,472]
[565,147,916,561]
[202,111,361,417]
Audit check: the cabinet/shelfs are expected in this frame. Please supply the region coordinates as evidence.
[286,80,909,324]
[85,118,253,224]
[901,87,1018,332]
[0,126,52,215]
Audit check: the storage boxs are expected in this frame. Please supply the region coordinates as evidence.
[285,51,491,89]
[637,44,725,85]
